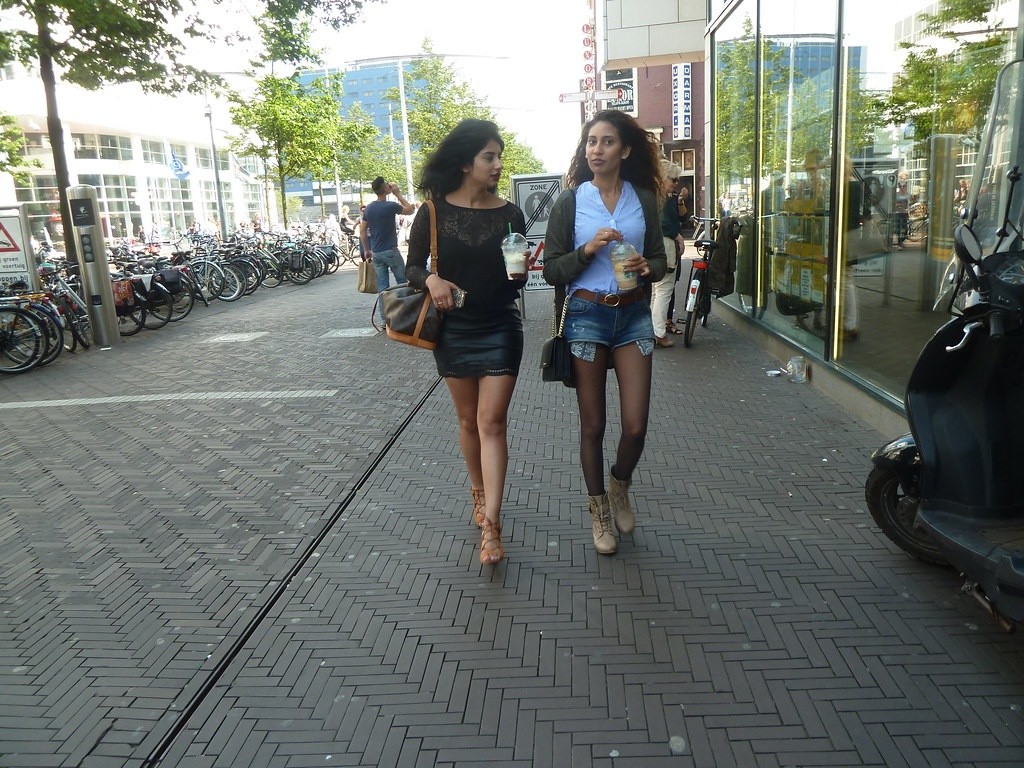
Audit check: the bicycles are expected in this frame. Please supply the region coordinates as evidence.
[0,253,91,375]
[105,220,264,337]
[261,227,363,288]
[677,214,742,347]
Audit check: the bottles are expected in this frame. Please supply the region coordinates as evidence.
[785,355,807,382]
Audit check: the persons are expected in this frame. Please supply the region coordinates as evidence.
[649,159,688,348]
[894,171,914,249]
[959,178,972,200]
[38,242,53,264]
[326,201,422,266]
[138,224,146,244]
[542,110,668,554]
[760,170,787,251]
[797,148,878,342]
[188,218,220,239]
[240,213,263,233]
[359,176,414,331]
[718,193,740,217]
[404,118,536,565]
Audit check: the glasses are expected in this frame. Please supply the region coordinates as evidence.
[667,177,679,183]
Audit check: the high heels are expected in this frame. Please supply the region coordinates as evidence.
[653,336,673,347]
[468,486,486,527]
[479,517,504,564]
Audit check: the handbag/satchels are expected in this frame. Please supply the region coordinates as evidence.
[356,259,377,295]
[369,198,442,350]
[539,335,571,383]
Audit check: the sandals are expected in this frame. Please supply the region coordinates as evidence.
[664,321,683,335]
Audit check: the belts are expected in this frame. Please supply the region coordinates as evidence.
[571,287,645,307]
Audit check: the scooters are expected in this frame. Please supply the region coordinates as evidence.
[863,223,1024,633]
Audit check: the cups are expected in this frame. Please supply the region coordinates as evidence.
[500,232,528,280]
[610,242,639,290]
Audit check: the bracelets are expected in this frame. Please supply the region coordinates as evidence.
[678,200,684,206]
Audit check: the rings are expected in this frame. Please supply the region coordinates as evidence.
[437,302,444,305]
[605,231,609,236]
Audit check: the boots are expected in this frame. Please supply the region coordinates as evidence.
[607,465,634,535]
[586,492,618,554]
